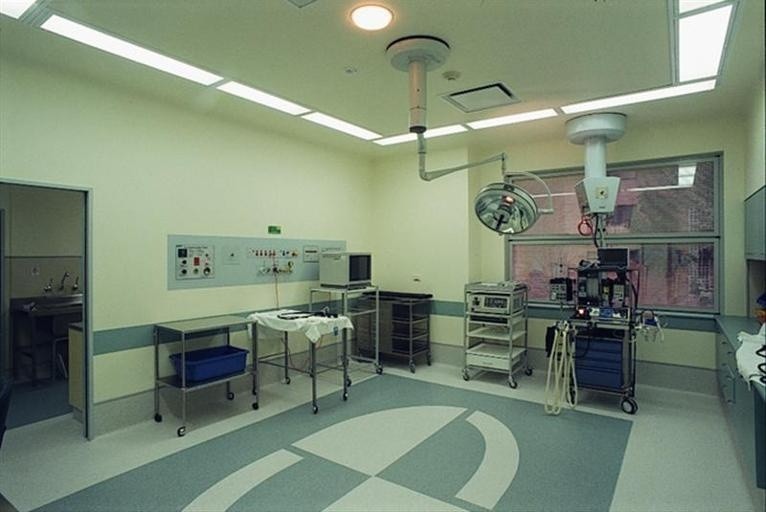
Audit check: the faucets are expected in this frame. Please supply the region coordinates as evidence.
[57,271,69,290]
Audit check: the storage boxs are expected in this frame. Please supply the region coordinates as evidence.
[169,344,250,382]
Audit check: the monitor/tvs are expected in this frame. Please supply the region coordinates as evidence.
[320,249,371,288]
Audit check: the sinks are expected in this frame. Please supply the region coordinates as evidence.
[44,294,82,306]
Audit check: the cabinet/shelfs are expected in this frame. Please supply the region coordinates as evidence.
[69,322,85,424]
[713,321,766,489]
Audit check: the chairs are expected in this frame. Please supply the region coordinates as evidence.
[743,188,765,262]
